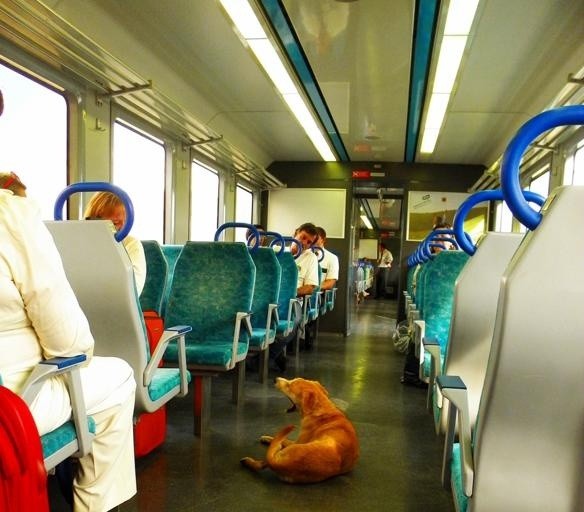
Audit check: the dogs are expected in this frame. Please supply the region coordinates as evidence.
[238,377,359,484]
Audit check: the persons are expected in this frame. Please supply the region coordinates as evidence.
[85,190,146,296]
[246,223,340,306]
[430,221,456,252]
[362,290,370,297]
[375,242,395,297]
[1,87,137,512]
[2,172,27,198]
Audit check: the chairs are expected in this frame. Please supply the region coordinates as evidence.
[437,104,584,512]
[402,189,547,411]
[43,182,193,413]
[132,232,258,437]
[0,354,97,476]
[161,219,340,404]
